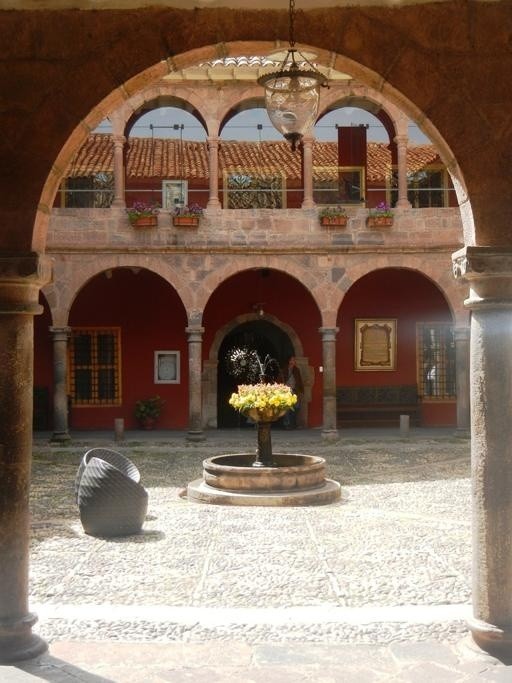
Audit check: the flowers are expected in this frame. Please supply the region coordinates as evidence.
[123,199,160,213]
[168,204,203,214]
[362,203,392,216]
[134,392,165,415]
[319,206,345,214]
[227,376,298,427]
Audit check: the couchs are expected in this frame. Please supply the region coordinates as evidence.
[335,383,422,428]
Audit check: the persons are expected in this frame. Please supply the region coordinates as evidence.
[277,354,304,430]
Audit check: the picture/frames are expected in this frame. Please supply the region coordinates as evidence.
[162,180,189,211]
[222,164,286,208]
[154,349,182,385]
[352,316,397,373]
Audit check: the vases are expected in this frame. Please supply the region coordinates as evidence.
[132,214,160,228]
[251,419,279,470]
[144,414,157,429]
[369,219,395,229]
[325,213,347,230]
[176,218,199,227]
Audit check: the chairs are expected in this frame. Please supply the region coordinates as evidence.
[76,447,142,496]
[80,457,147,537]
[33,385,52,431]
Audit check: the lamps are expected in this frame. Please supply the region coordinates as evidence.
[254,0,332,150]
